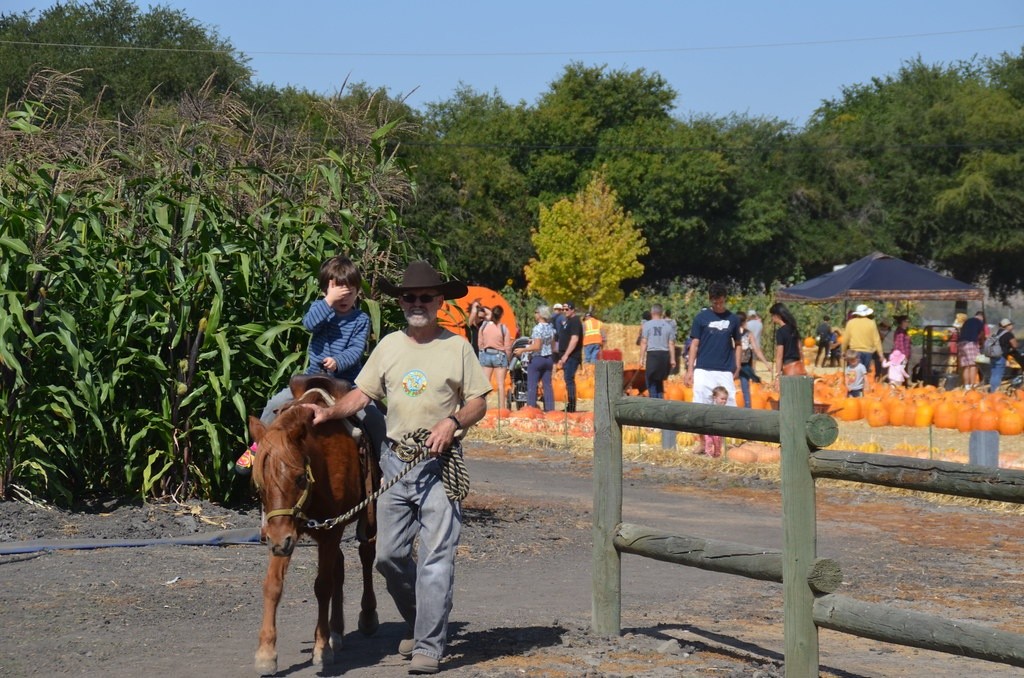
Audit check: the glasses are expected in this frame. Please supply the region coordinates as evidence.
[400,293,441,303]
[535,312,539,314]
[561,309,569,312]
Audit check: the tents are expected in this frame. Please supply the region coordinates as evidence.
[773,249,987,381]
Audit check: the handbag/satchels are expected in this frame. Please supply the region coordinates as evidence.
[783,360,807,375]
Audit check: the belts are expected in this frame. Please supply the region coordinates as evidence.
[384,438,413,454]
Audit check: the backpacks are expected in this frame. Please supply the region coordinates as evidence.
[983,329,1009,359]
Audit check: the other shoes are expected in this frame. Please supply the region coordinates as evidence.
[408,654,439,673]
[398,632,414,656]
[236,443,263,474]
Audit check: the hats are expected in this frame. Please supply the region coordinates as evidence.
[890,350,905,365]
[553,303,563,308]
[563,302,576,309]
[999,319,1015,328]
[583,312,592,321]
[747,309,757,317]
[378,262,468,300]
[851,305,873,316]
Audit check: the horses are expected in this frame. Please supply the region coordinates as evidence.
[248,373,379,675]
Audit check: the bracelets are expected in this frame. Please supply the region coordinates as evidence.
[448,415,461,430]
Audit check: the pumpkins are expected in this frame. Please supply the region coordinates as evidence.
[476,363,594,439]
[624,330,1024,453]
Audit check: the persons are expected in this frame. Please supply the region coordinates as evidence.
[813,303,1024,399]
[462,300,609,412]
[234,255,385,476]
[294,261,494,674]
[636,286,811,458]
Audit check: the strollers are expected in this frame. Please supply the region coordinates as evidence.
[506,336,544,412]
